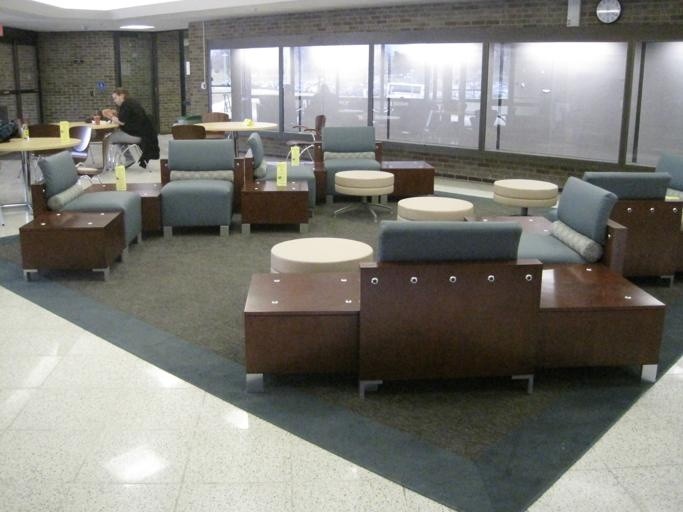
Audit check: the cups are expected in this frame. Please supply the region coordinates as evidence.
[277,162,287,187]
[93,116,101,125]
[115,165,126,190]
[60,121,70,143]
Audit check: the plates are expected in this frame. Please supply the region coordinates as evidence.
[91,120,111,124]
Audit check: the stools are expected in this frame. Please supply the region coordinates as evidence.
[269,238,374,275]
[333,170,394,222]
[494,179,558,216]
[397,196,474,221]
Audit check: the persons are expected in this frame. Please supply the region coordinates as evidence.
[90,87,147,179]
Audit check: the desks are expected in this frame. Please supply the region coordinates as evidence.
[239,180,310,235]
[244,272,361,392]
[85,183,163,235]
[381,160,435,204]
[194,121,278,157]
[19,212,126,281]
[50,121,120,129]
[0,137,82,216]
[536,263,667,381]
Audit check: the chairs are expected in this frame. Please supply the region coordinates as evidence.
[464,176,628,278]
[234,131,317,207]
[28,124,66,157]
[314,125,381,203]
[31,151,143,261]
[202,113,233,139]
[360,220,543,398]
[547,171,682,278]
[160,138,236,240]
[286,115,326,161]
[68,125,92,160]
[172,125,206,140]
[109,142,153,172]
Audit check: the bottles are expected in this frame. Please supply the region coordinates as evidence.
[21,121,29,141]
[291,147,299,165]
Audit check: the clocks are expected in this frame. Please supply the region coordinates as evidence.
[595,0,621,24]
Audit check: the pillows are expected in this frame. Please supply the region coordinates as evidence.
[323,151,375,160]
[169,170,235,182]
[548,219,602,263]
[666,187,682,200]
[48,176,94,211]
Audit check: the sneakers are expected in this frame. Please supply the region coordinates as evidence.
[125,160,135,168]
